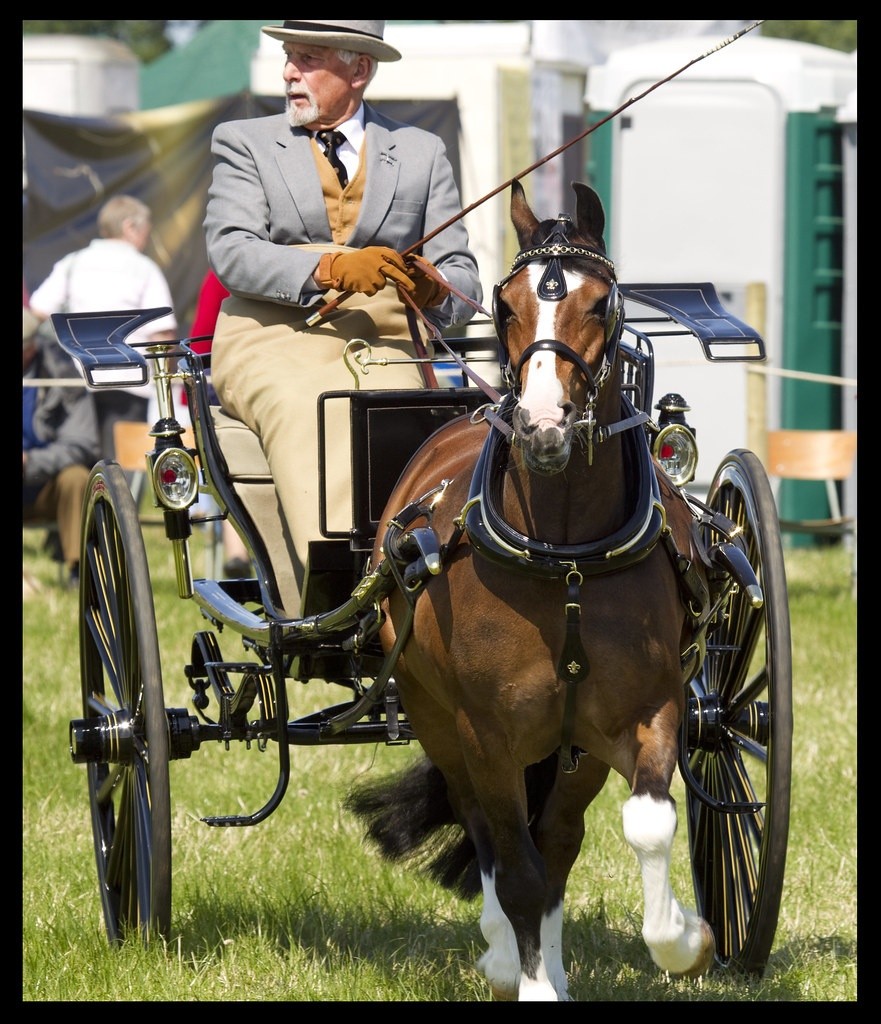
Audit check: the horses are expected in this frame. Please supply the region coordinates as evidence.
[369,179,718,1001]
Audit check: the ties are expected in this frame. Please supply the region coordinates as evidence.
[318,131,349,190]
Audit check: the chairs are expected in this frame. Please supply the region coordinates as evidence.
[183,333,304,620]
[768,431,857,599]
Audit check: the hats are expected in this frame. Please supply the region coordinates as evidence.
[261,20,401,62]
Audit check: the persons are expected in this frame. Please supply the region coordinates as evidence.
[23,194,176,580]
[202,20,485,567]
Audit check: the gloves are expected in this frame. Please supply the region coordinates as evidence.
[396,253,450,310]
[319,246,415,297]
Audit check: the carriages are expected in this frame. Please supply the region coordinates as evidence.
[67,175,799,978]
